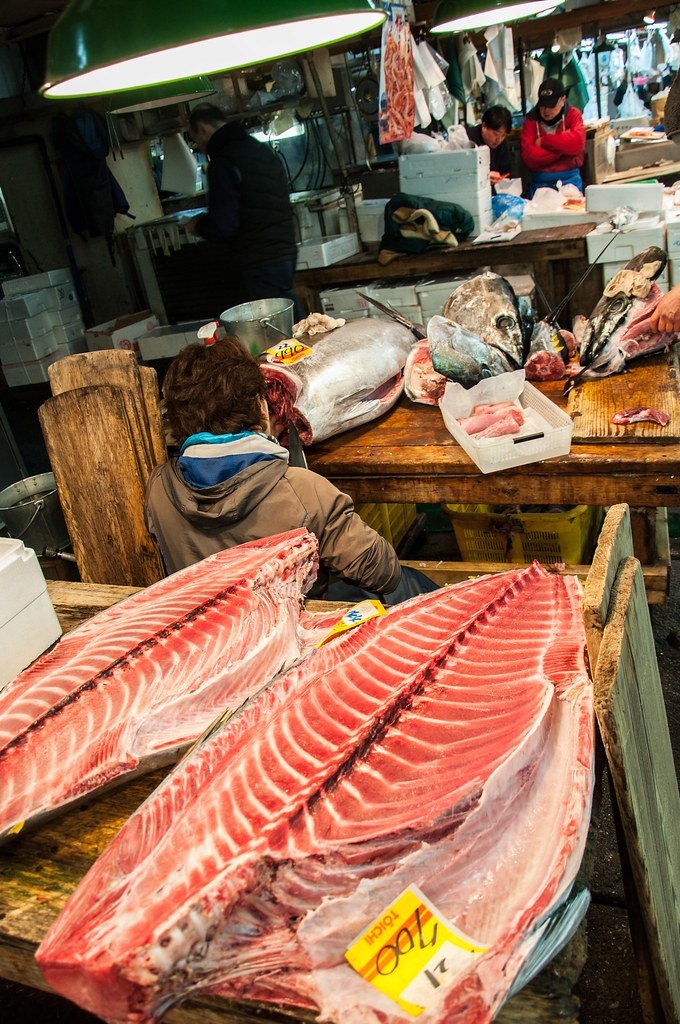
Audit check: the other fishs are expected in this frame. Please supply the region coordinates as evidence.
[578,247,669,368]
[427,314,514,390]
[442,271,526,369]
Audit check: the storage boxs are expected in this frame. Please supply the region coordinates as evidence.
[665,220,680,260]
[602,262,669,288]
[355,502,418,550]
[437,379,573,474]
[443,502,593,564]
[584,182,666,212]
[581,129,616,185]
[586,217,666,264]
[0,265,215,387]
[613,116,650,135]
[670,260,680,287]
[318,267,476,325]
[338,144,493,243]
[296,233,360,270]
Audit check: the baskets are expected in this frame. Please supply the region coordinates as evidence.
[438,381,574,474]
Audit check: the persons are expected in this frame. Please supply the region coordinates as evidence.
[145,330,441,607]
[176,101,305,323]
[514,76,586,195]
[462,106,524,200]
[648,281,680,336]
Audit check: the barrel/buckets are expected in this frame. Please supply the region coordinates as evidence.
[219,298,294,359]
[0,471,69,557]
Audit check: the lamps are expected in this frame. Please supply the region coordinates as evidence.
[107,76,218,117]
[37,0,390,101]
[642,10,657,23]
[427,0,566,34]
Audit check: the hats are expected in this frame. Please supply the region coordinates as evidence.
[537,78,565,109]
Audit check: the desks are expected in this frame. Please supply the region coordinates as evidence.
[315,397,680,605]
[0,579,605,1024]
[292,221,592,320]
